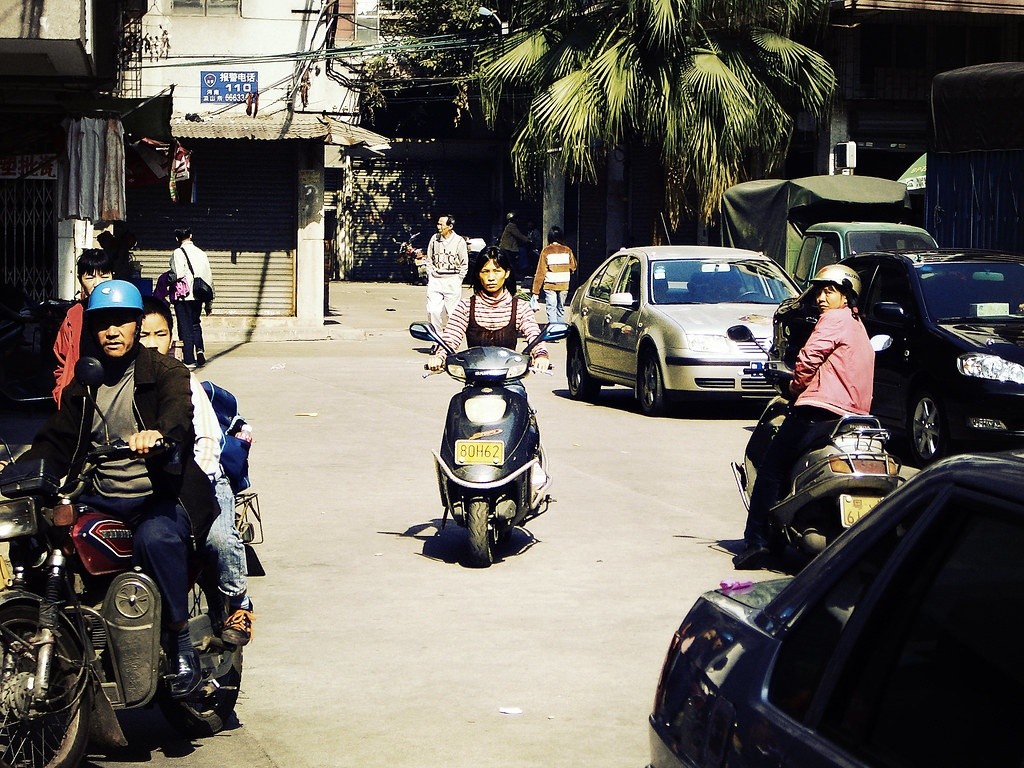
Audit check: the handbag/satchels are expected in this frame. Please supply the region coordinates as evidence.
[530,295,540,312]
[193,277,213,303]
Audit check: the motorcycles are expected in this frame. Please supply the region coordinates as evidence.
[392,227,429,285]
[0,356,265,768]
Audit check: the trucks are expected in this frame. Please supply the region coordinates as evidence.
[718,175,939,300]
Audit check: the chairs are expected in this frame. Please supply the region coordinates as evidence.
[653,278,676,304]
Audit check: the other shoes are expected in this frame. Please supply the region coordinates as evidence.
[730,545,770,571]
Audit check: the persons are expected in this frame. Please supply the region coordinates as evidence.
[658,626,732,735]
[498,214,544,296]
[170,226,214,370]
[531,226,578,343]
[732,265,875,570]
[0,279,254,699]
[52,247,115,410]
[175,151,192,204]
[425,215,469,355]
[428,247,549,492]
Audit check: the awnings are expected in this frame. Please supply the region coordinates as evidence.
[101,93,174,145]
[170,117,331,140]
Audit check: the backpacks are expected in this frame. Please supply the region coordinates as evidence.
[201,381,253,494]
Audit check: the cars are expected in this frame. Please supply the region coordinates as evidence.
[646,454,1024,768]
[763,249,1024,467]
[565,244,804,415]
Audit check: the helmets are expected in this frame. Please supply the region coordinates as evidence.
[85,280,143,312]
[810,265,862,296]
[507,212,516,219]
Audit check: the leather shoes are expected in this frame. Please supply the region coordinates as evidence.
[168,646,202,697]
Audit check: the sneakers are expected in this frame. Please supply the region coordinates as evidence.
[222,598,256,646]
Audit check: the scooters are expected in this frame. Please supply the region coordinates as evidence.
[471,235,540,285]
[725,324,907,575]
[408,321,572,569]
[0,283,88,419]
